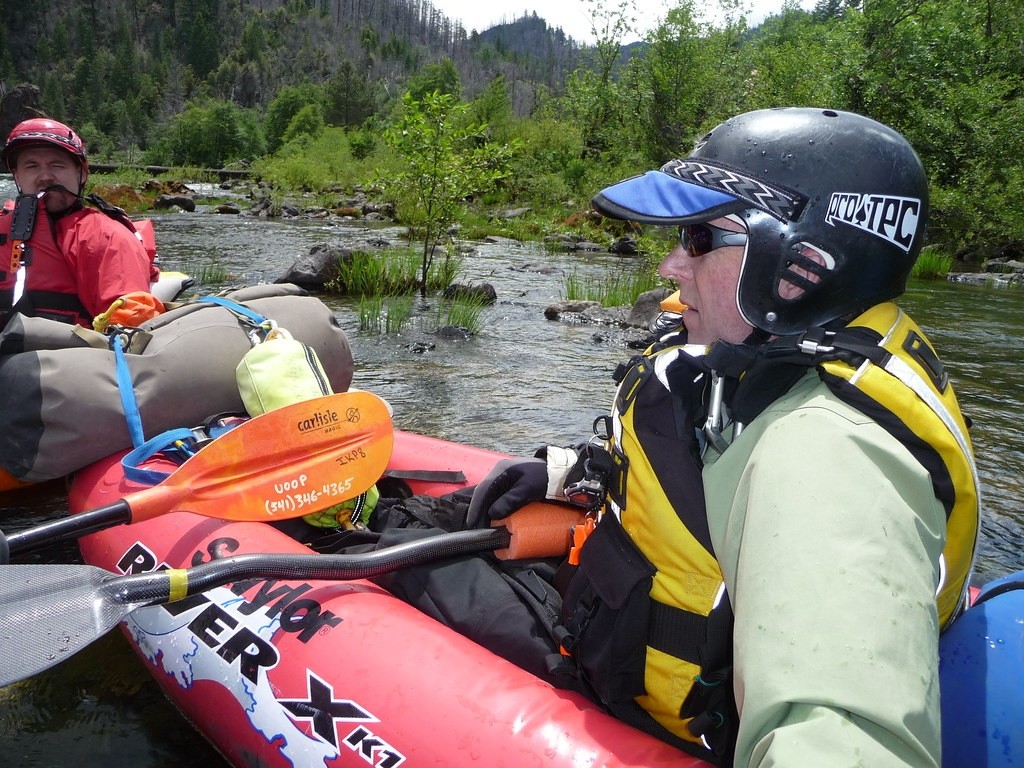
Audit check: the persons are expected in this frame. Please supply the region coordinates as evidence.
[0,118,167,333]
[302,106,984,768]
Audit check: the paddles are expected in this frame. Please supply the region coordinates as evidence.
[0,525,516,689]
[0,390,393,550]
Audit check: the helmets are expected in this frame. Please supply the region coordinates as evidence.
[0,118,87,156]
[591,106,929,336]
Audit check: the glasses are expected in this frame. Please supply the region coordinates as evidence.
[677,222,749,258]
